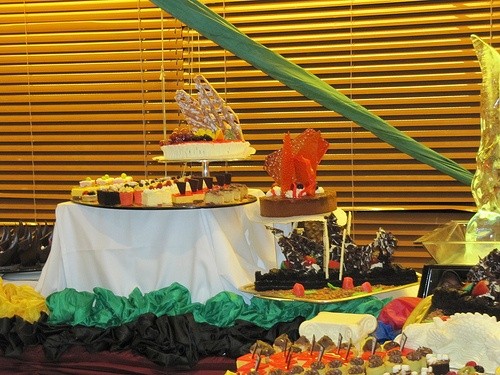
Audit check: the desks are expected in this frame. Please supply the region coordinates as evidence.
[40,186,293,310]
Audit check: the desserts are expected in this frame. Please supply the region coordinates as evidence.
[223,332,500,375]
[71,173,248,205]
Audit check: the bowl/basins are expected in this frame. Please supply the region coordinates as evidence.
[414,220,500,266]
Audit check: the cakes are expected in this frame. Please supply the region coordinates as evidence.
[254,213,419,291]
[160,75,250,159]
[431,248,500,322]
[259,128,338,217]
